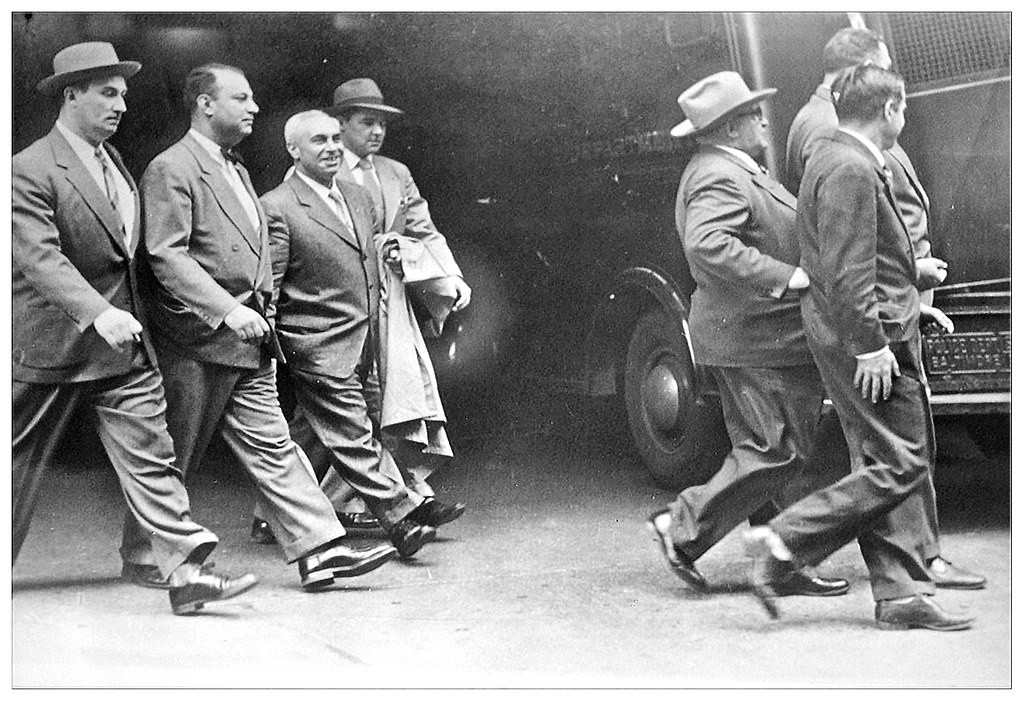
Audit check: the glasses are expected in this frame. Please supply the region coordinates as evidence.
[723,106,763,124]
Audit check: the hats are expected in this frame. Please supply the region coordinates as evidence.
[325,78,401,113]
[38,42,143,97]
[670,70,775,137]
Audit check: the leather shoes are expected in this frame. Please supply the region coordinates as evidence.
[773,571,850,596]
[392,518,435,561]
[876,595,975,632]
[250,517,277,544]
[299,544,397,587]
[647,508,705,593]
[927,556,987,588]
[121,561,174,587]
[335,509,381,529]
[411,500,469,529]
[170,559,257,615]
[737,528,782,623]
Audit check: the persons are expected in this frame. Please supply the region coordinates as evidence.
[118,66,397,589]
[13,42,258,613]
[787,27,987,589]
[287,78,471,528]
[742,59,976,631]
[252,112,436,558]
[649,68,850,596]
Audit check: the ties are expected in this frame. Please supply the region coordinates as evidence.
[95,149,127,239]
[360,160,385,228]
[329,191,357,239]
[758,165,771,178]
[884,165,895,193]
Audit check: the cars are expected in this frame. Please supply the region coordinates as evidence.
[364,12,1010,494]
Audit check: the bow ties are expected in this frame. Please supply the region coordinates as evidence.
[220,147,237,167]
